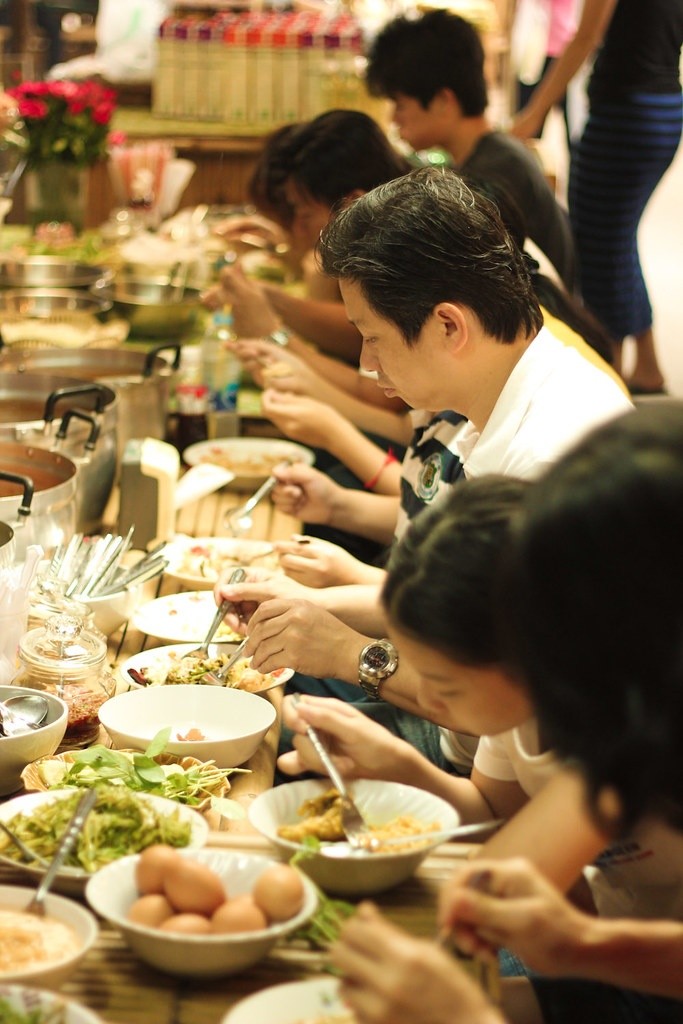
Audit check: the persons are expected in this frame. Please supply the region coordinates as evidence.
[512,0,683,395]
[367,8,579,298]
[216,108,683,1024]
[502,0,586,159]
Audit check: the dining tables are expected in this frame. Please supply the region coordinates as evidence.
[0,210,504,1024]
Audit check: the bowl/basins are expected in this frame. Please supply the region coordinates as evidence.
[0,790,210,892]
[247,776,461,892]
[83,846,319,975]
[80,565,145,637]
[119,643,295,693]
[0,883,100,990]
[152,537,303,590]
[24,748,232,814]
[1,683,70,798]
[96,682,278,767]
[94,279,204,339]
[0,983,106,1024]
[182,436,316,490]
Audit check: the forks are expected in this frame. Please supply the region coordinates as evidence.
[289,691,374,848]
[0,702,43,737]
[199,636,251,686]
[180,569,246,657]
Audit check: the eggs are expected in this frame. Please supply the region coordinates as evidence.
[127,843,303,937]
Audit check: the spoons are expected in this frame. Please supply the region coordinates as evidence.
[0,694,48,726]
[225,459,293,533]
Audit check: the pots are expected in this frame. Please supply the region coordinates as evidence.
[1,254,170,572]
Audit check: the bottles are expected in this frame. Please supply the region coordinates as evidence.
[166,309,240,466]
[10,617,117,747]
[155,1,396,134]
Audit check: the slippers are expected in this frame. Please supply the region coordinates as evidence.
[622,374,667,394]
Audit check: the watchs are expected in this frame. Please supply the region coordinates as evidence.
[358,638,398,699]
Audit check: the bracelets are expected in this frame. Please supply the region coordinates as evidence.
[365,452,397,488]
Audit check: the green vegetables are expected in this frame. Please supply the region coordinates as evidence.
[286,834,356,977]
[0,727,255,876]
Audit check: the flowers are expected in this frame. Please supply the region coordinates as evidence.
[0,77,116,159]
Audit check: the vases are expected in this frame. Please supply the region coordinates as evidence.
[21,157,91,232]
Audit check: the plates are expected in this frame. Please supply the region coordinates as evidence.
[221,974,356,1024]
[133,590,248,643]
